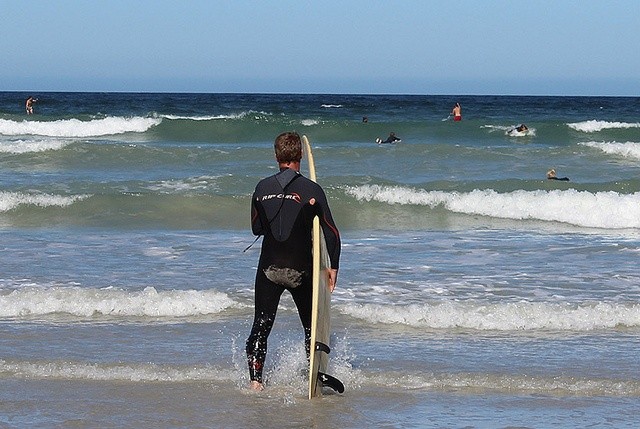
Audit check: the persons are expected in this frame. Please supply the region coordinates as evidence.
[547,169,556,178]
[25,96,38,114]
[247,133,345,393]
[375,131,401,144]
[450,102,462,121]
[508,125,527,133]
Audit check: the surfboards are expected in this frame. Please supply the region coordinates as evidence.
[299,136,345,399]
